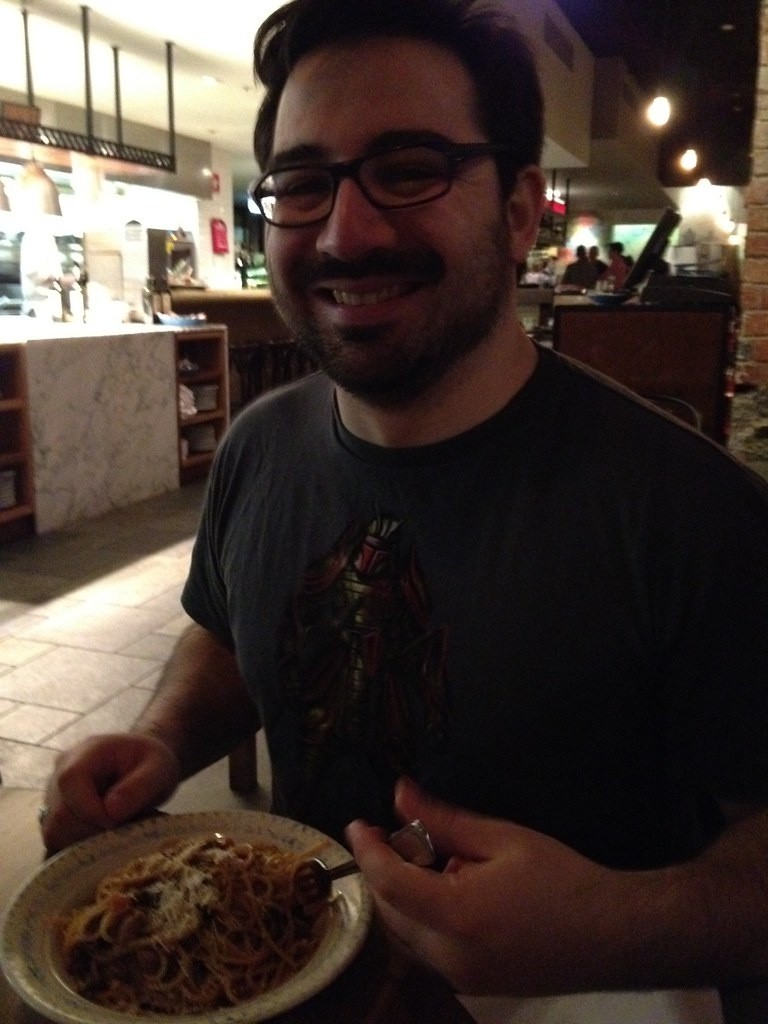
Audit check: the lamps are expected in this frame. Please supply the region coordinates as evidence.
[10,9,61,216]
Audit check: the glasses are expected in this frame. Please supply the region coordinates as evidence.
[251,141,529,228]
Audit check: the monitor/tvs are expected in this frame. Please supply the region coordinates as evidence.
[620,207,682,288]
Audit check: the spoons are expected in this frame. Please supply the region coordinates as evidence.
[291,817,434,908]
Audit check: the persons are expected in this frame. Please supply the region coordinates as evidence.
[525,260,546,283]
[606,242,627,274]
[564,245,598,287]
[40,0,768,1024]
[588,245,608,273]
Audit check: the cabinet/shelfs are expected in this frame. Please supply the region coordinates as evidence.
[0,343,34,521]
[175,325,231,475]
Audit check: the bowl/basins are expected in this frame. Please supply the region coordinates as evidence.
[159,316,207,328]
[588,293,635,307]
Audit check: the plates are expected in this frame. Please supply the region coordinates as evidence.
[0,808,374,1024]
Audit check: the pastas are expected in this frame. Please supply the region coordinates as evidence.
[54,831,331,1016]
